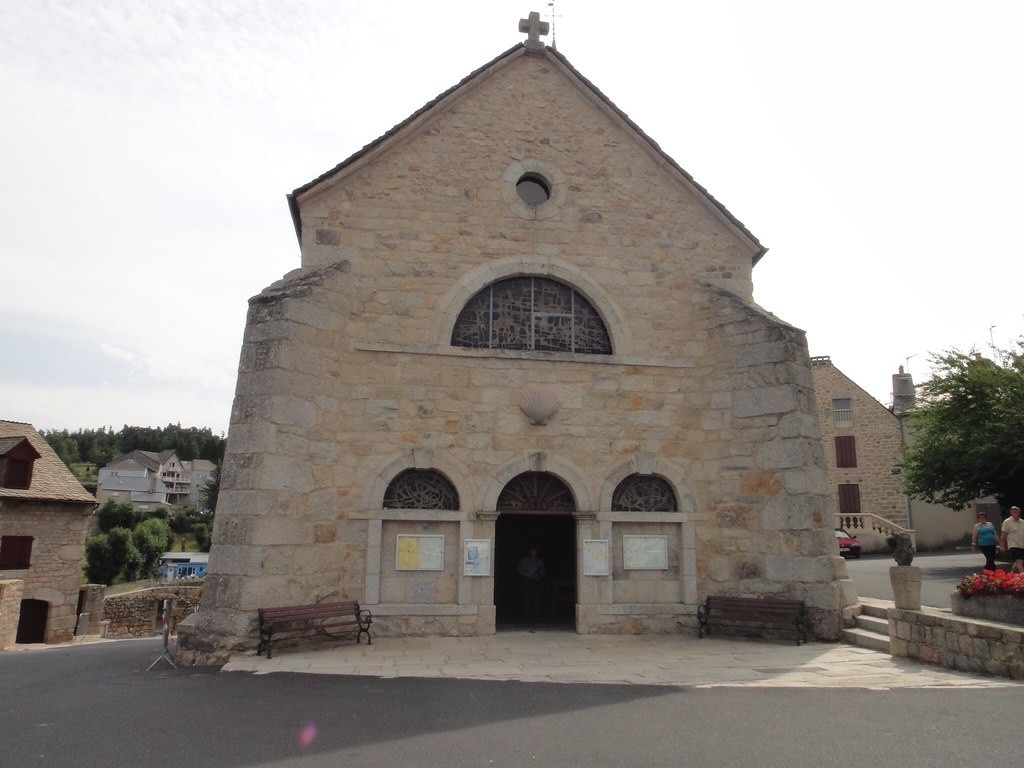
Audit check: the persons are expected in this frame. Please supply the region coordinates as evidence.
[972,512,1001,570]
[1000,506,1024,574]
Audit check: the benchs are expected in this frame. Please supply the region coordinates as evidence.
[696,595,808,647]
[256,600,373,660]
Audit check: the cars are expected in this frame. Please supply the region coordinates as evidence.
[834,528,861,559]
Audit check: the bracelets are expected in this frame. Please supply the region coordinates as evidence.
[972,543,976,545]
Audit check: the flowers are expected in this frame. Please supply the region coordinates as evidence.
[956,568,1024,597]
